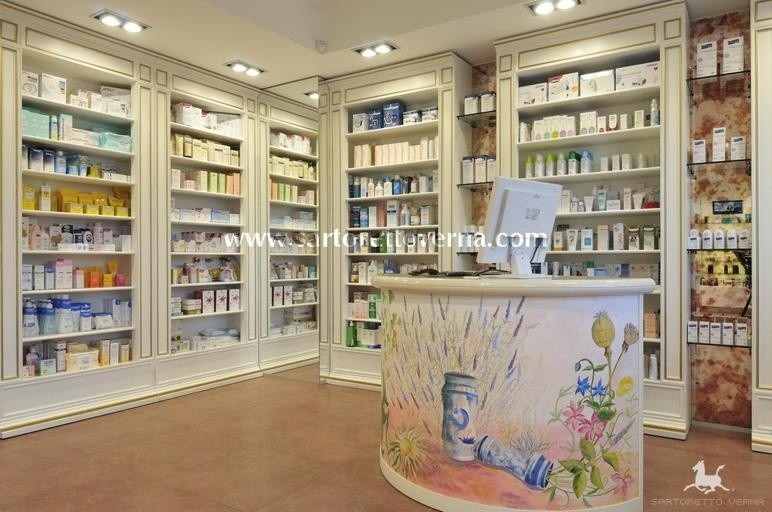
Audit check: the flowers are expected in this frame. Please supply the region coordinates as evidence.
[548,314,641,503]
[384,421,437,478]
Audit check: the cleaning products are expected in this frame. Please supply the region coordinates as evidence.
[22,293,73,338]
[524,149,593,179]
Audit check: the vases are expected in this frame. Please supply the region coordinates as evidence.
[475,436,555,490]
[440,371,481,463]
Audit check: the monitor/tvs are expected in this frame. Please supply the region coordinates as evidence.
[476,175,563,279]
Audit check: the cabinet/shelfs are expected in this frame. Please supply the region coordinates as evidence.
[456,111,505,254]
[691,69,753,350]
[326,55,472,392]
[750,0,772,451]
[255,89,322,373]
[494,0,688,443]
[0,0,153,442]
[154,51,256,398]
